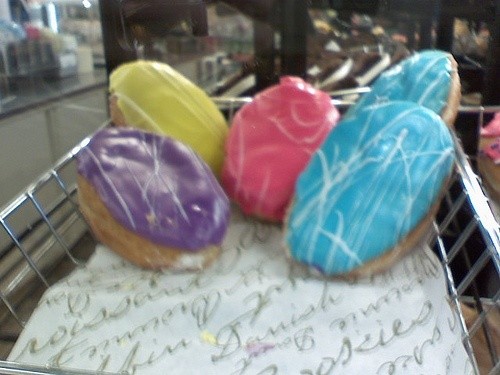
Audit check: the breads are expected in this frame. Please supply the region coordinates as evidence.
[283,101,455,281]
[75,129,231,273]
[222,76,339,222]
[108,57,228,177]
[344,49,460,124]
